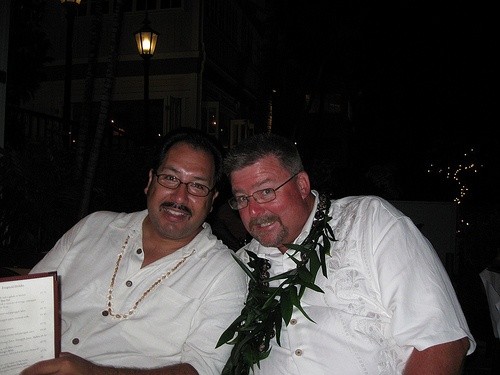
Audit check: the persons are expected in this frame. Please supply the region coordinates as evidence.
[222,132,477,375]
[19,130,248,375]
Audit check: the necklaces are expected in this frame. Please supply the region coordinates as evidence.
[215,194,338,375]
[107,235,194,318]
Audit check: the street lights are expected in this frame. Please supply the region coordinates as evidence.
[58,0,82,233]
[134,10,158,209]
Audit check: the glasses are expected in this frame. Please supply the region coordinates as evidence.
[227,169,303,211]
[155,170,214,198]
